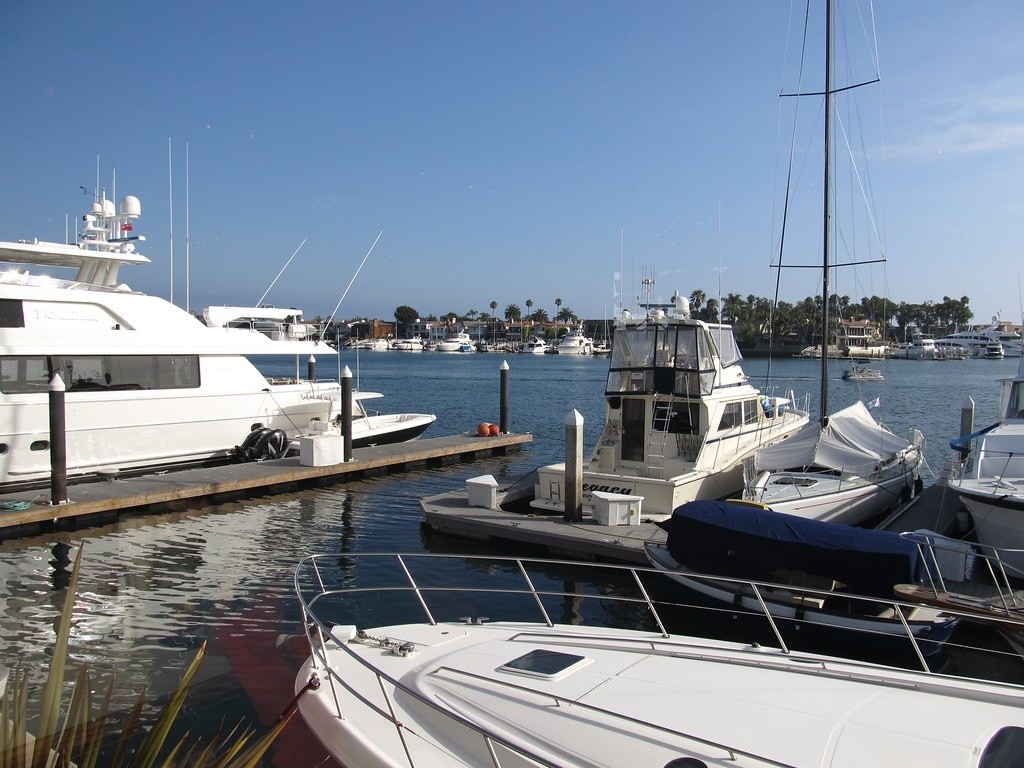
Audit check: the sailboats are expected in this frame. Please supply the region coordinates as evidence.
[593,304,612,356]
[741,0,925,527]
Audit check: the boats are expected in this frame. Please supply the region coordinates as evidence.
[946,321,1024,591]
[392,337,426,350]
[460,344,477,352]
[293,551,1024,768]
[643,501,961,673]
[285,414,437,457]
[435,329,472,352]
[521,335,553,354]
[363,339,388,349]
[842,367,885,382]
[556,325,594,355]
[892,532,1024,663]
[427,342,437,351]
[791,317,1024,360]
[0,137,384,494]
[527,226,811,522]
